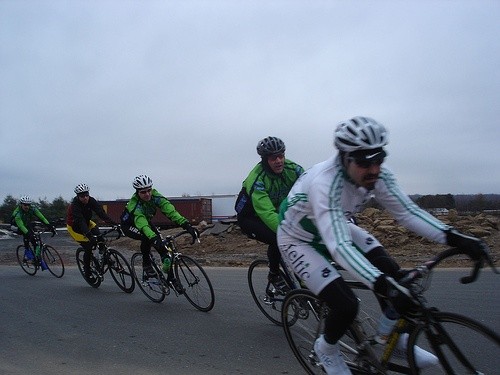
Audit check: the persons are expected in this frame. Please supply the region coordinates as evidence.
[67,184,119,284]
[277,118,489,375]
[234,136,308,292]
[12,195,57,271]
[124,175,200,294]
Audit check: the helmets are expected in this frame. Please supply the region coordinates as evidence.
[73,183,90,193]
[256,136,286,155]
[132,174,154,189]
[333,116,391,152]
[20,196,32,204]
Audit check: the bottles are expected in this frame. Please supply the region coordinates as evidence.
[99,250,104,265]
[163,257,171,273]
[35,244,41,257]
[374,307,400,346]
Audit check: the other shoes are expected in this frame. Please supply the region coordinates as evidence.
[268,269,295,296]
[171,277,183,293]
[100,258,115,265]
[142,262,157,278]
[85,272,96,283]
[40,261,47,271]
[24,250,34,259]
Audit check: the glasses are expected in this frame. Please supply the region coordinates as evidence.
[140,188,152,194]
[79,193,89,198]
[24,204,30,206]
[266,153,285,160]
[346,151,387,168]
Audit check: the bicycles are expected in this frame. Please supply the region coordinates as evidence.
[251,243,375,329]
[76,224,136,293]
[281,246,500,375]
[132,224,217,312]
[16,223,65,278]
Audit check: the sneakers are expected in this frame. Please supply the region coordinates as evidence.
[314,334,353,375]
[374,318,439,369]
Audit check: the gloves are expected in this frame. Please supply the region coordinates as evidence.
[86,232,98,245]
[372,272,422,321]
[182,221,201,239]
[446,228,486,262]
[151,235,163,248]
